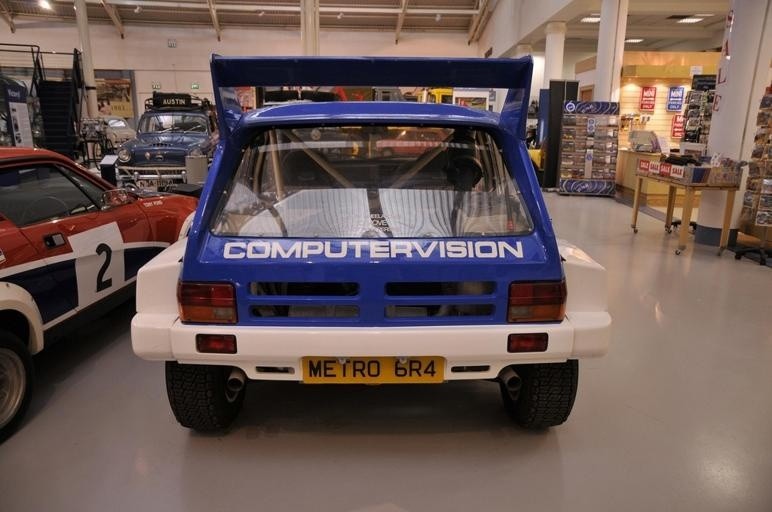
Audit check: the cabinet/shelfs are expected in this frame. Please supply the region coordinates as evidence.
[558,99,620,197]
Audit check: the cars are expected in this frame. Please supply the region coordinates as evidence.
[97,115,138,150]
[129,49,620,436]
[372,129,449,158]
[523,123,542,170]
[0,144,203,447]
[113,90,222,192]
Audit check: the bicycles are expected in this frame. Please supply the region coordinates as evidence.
[92,125,115,172]
